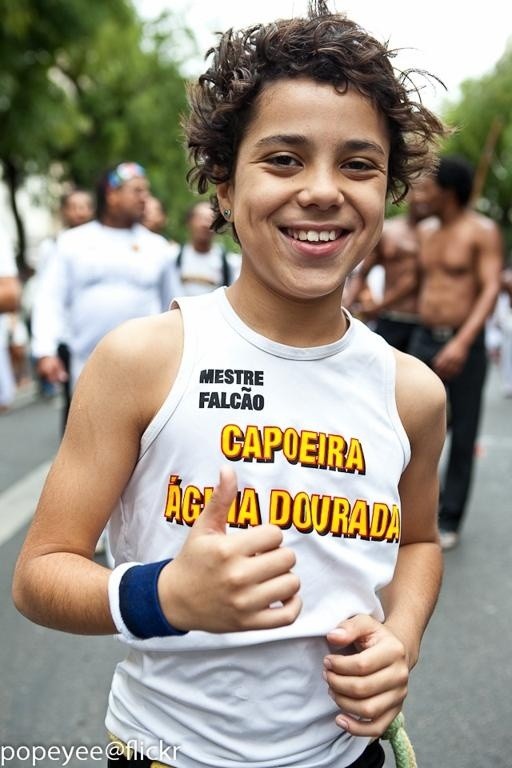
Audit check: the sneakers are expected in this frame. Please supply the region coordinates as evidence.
[437,531,458,550]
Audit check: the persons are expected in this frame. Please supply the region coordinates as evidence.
[176,198,244,297]
[7,3,458,766]
[493,268,511,399]
[1,185,24,414]
[61,189,94,228]
[356,155,507,554]
[143,194,169,233]
[342,167,433,353]
[26,162,179,440]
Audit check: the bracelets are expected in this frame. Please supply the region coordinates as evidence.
[107,557,190,643]
